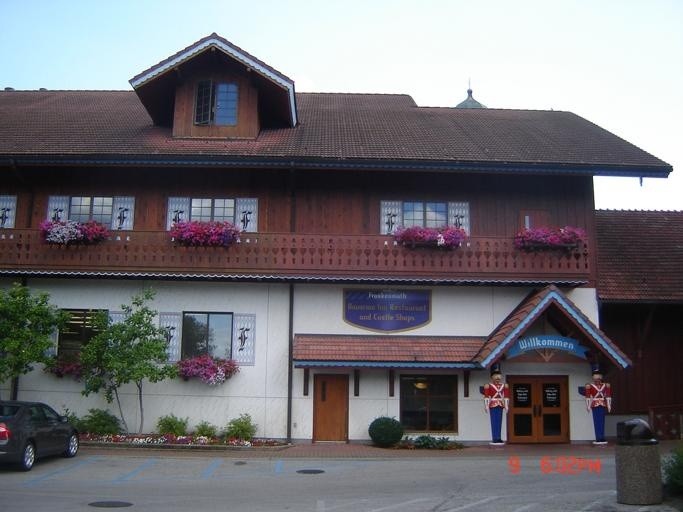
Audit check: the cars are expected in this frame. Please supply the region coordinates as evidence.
[1,400,81,472]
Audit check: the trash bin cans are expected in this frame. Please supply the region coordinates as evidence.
[615,418,664,505]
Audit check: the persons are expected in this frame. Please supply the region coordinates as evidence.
[483,363,511,443]
[584,363,613,441]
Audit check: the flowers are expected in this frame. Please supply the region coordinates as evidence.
[515,225,588,250]
[392,224,468,250]
[42,361,103,381]
[40,219,111,244]
[170,221,241,245]
[176,352,240,386]
